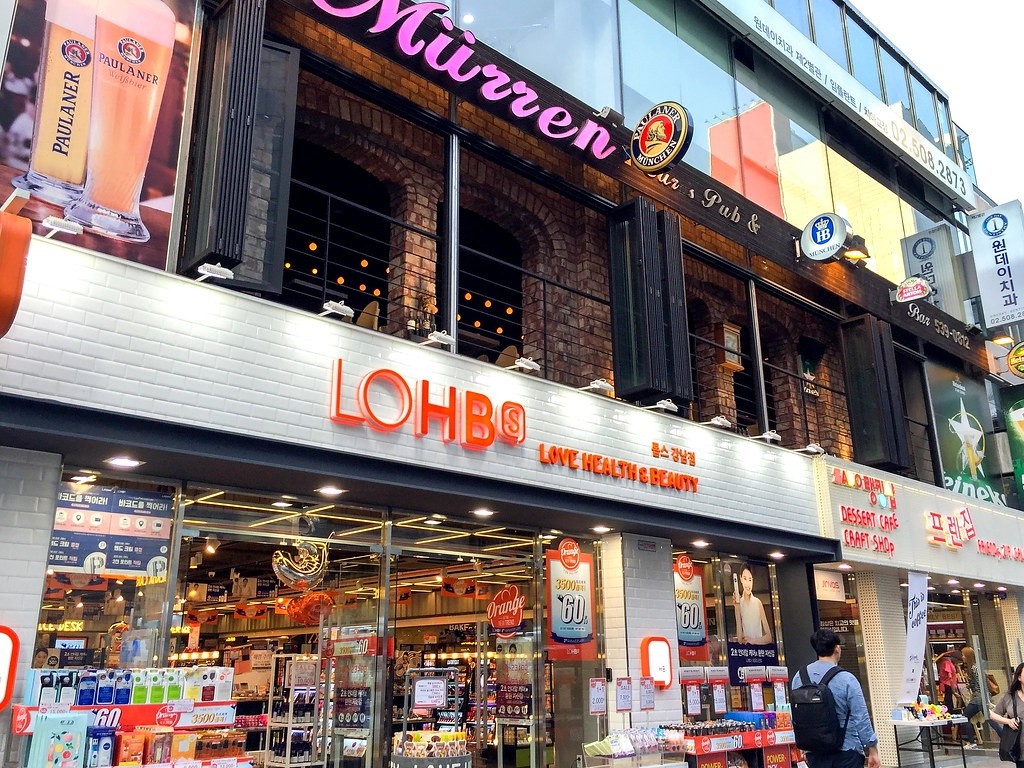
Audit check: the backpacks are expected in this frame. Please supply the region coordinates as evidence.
[789,662,850,755]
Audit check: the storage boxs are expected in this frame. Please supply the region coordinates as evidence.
[83,726,116,768]
[115,733,145,766]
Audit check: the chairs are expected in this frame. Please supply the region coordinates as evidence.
[476,346,519,371]
[342,301,380,330]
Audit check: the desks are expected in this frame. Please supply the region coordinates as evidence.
[891,717,968,768]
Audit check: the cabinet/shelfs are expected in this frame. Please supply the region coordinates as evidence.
[232,696,284,731]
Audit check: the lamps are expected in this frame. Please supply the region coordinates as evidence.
[195,262,234,281]
[593,107,625,126]
[475,559,483,574]
[578,378,614,391]
[208,539,221,553]
[793,443,824,454]
[419,330,456,346]
[505,357,540,370]
[642,399,678,412]
[356,581,362,590]
[845,235,870,259]
[41,215,83,238]
[965,324,982,336]
[190,557,197,568]
[699,416,731,428]
[750,430,781,441]
[318,300,354,317]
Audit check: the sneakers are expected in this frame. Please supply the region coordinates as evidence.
[963,743,978,751]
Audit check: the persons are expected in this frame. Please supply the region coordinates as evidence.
[959,647,1003,749]
[508,644,527,684]
[189,584,202,600]
[939,653,960,715]
[792,629,881,768]
[989,663,1024,768]
[34,648,48,668]
[732,563,772,645]
[239,579,252,596]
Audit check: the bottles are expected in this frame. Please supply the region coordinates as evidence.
[269,686,319,763]
[636,726,684,751]
[903,701,947,720]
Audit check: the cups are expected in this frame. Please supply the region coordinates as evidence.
[11,0,176,244]
[948,438,980,481]
[1008,398,1024,440]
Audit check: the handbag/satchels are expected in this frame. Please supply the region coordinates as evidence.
[999,690,1021,763]
[985,672,1000,696]
